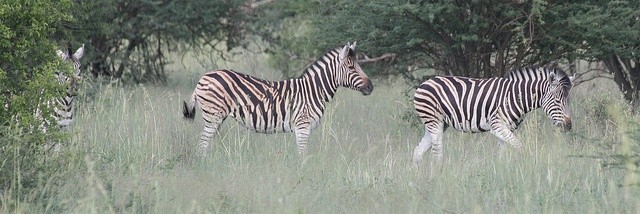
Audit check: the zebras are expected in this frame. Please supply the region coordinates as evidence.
[5,44,84,141]
[413,66,577,162]
[182,41,373,154]
